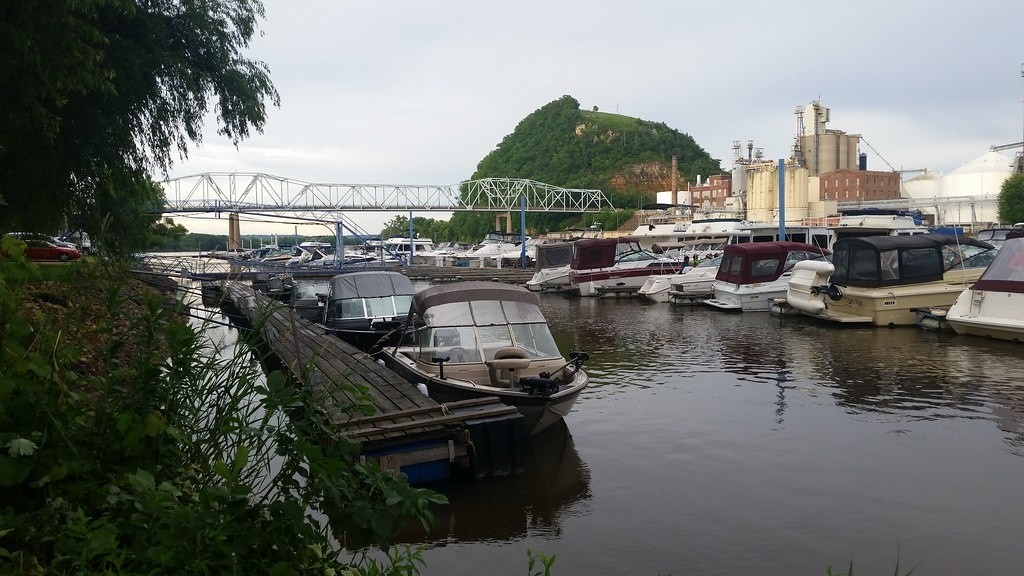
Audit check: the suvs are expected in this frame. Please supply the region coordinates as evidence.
[8,231,78,249]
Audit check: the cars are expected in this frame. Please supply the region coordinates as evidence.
[21,239,77,262]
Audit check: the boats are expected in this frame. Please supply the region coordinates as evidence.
[638,255,725,305]
[909,305,955,333]
[247,271,324,323]
[316,272,424,355]
[523,206,950,297]
[765,297,803,318]
[226,228,605,271]
[702,241,838,313]
[787,233,1000,326]
[380,279,591,439]
[944,226,1024,343]
